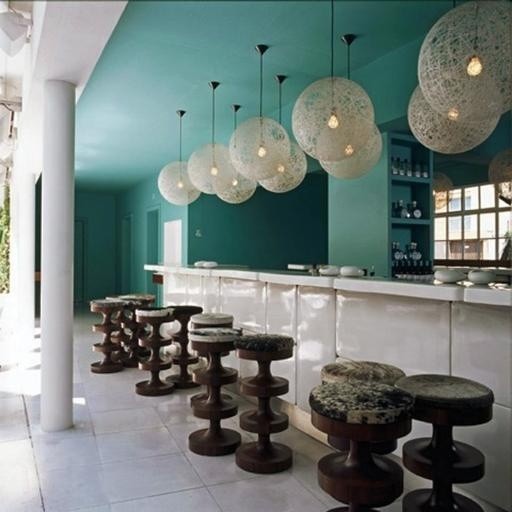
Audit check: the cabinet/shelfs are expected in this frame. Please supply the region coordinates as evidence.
[326,132,435,280]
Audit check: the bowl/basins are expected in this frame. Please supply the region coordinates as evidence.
[194,260,218,269]
[319,265,358,277]
[434,269,497,285]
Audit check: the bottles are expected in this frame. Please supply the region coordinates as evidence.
[370,264,376,277]
[391,156,429,178]
[395,199,423,219]
[391,241,433,276]
